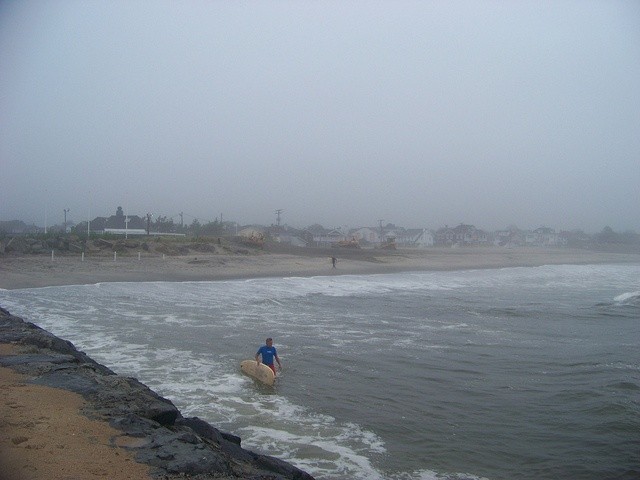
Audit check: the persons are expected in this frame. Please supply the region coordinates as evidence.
[255,338,282,376]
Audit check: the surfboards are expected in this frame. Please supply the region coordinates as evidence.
[240,360,274,386]
